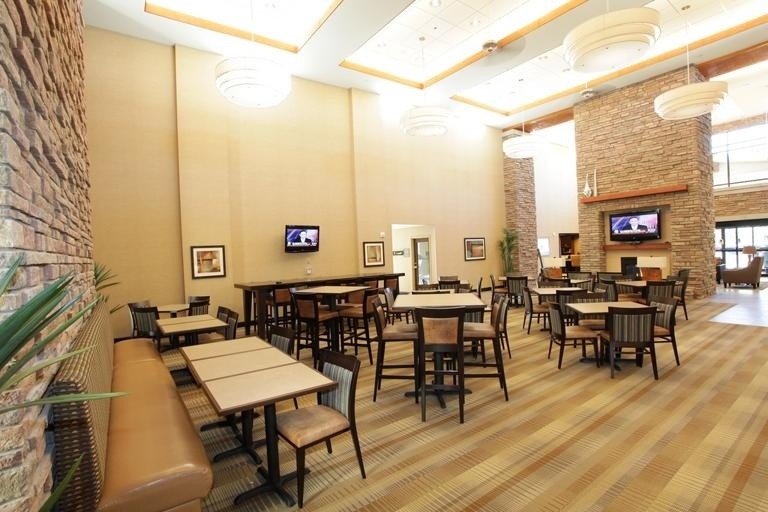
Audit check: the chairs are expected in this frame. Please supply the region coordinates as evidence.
[721,255,764,290]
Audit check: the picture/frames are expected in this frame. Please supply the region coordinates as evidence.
[187,244,227,281]
[462,235,488,262]
[362,240,387,269]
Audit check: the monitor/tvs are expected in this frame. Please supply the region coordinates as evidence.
[284,224,320,253]
[608,211,661,241]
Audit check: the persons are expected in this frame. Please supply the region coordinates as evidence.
[621,216,648,233]
[295,229,313,245]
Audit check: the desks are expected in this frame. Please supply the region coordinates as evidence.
[232,273,407,336]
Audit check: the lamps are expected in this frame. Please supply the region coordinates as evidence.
[741,245,756,265]
[558,0,729,124]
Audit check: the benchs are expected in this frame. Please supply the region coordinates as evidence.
[47,298,216,512]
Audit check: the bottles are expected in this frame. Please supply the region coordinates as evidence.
[304,258,312,278]
